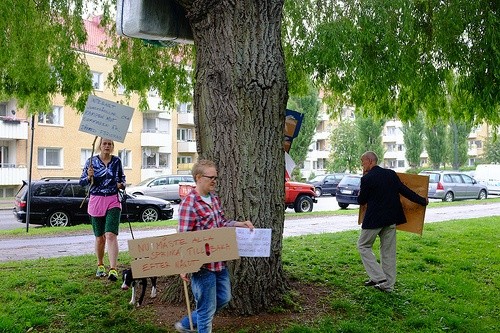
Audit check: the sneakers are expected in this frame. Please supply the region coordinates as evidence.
[108,270,118,282]
[96,265,106,277]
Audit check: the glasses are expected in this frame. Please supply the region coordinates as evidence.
[201,175,218,181]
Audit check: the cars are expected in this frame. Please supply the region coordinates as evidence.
[305,174,362,209]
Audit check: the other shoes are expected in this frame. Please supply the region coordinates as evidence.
[365,278,386,286]
[374,285,384,292]
[175,322,190,333]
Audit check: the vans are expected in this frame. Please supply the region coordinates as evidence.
[474,164,500,195]
[124,174,196,201]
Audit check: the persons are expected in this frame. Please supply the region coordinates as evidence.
[356,151,429,292]
[174,160,254,333]
[78,136,126,280]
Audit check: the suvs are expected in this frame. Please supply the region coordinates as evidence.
[416,169,488,202]
[12,176,174,228]
[285,181,318,213]
